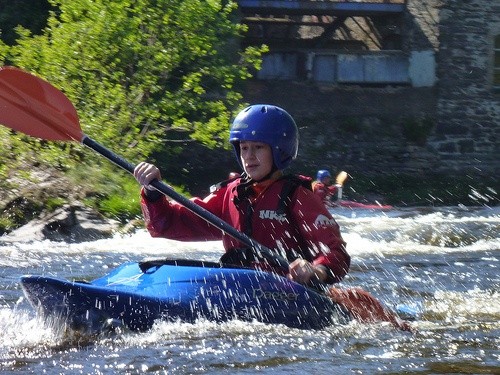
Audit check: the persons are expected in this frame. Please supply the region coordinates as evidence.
[312,168,340,199]
[133,104,350,291]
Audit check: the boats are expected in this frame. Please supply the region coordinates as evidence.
[17,257,418,341]
[336,199,392,213]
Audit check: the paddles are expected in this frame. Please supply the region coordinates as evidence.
[0,66,398,325]
[336,171,348,200]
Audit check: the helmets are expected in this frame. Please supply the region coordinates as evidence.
[317,169,331,180]
[228,103,300,170]
